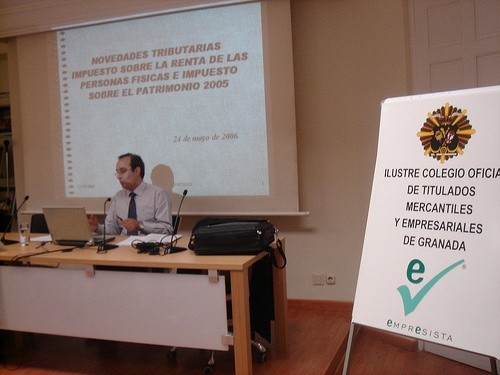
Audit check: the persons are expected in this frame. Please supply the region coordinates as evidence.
[88,153,175,235]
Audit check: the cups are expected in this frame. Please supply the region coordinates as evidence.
[19,223,30,246]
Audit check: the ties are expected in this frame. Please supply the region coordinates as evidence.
[127,192,138,235]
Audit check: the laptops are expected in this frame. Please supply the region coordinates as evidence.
[41,206,115,246]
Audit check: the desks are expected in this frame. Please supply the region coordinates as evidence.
[0,231,287,375]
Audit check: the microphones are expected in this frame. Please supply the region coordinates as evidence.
[155,189,188,254]
[98,197,118,251]
[0,194,29,246]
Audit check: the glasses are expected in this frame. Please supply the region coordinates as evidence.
[114,168,132,175]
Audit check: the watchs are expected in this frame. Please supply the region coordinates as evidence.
[138,220,145,231]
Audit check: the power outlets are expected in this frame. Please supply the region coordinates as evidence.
[312,274,324,284]
[326,274,336,284]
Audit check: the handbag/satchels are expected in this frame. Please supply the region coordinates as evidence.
[189,218,286,269]
